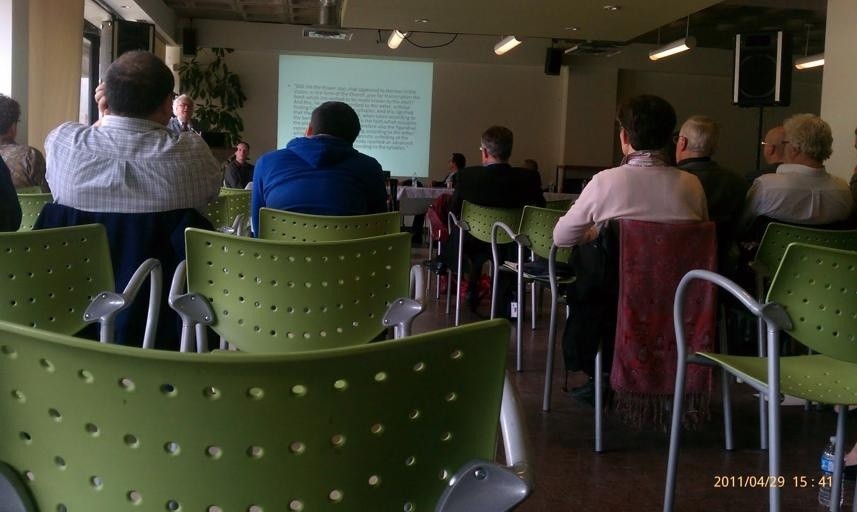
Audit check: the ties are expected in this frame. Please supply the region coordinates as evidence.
[182,122,189,131]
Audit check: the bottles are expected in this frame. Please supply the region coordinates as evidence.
[818,436,845,507]
[549,182,554,192]
[412,172,417,188]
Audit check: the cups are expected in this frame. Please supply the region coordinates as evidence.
[447,182,452,189]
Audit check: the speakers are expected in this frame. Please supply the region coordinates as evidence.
[544,46,563,77]
[98,18,156,78]
[729,27,794,109]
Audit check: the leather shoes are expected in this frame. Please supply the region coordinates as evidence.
[572,380,608,408]
[424,259,447,275]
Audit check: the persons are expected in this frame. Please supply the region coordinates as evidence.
[441,152,541,191]
[742,111,853,227]
[551,92,710,408]
[167,93,215,152]
[744,124,787,181]
[420,125,548,304]
[1,154,22,234]
[671,115,754,230]
[224,142,254,190]
[43,50,221,214]
[250,99,387,241]
[846,128,857,235]
[1,93,46,189]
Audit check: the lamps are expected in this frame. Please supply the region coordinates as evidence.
[794,23,824,70]
[387,30,408,50]
[648,12,697,62]
[493,36,523,57]
